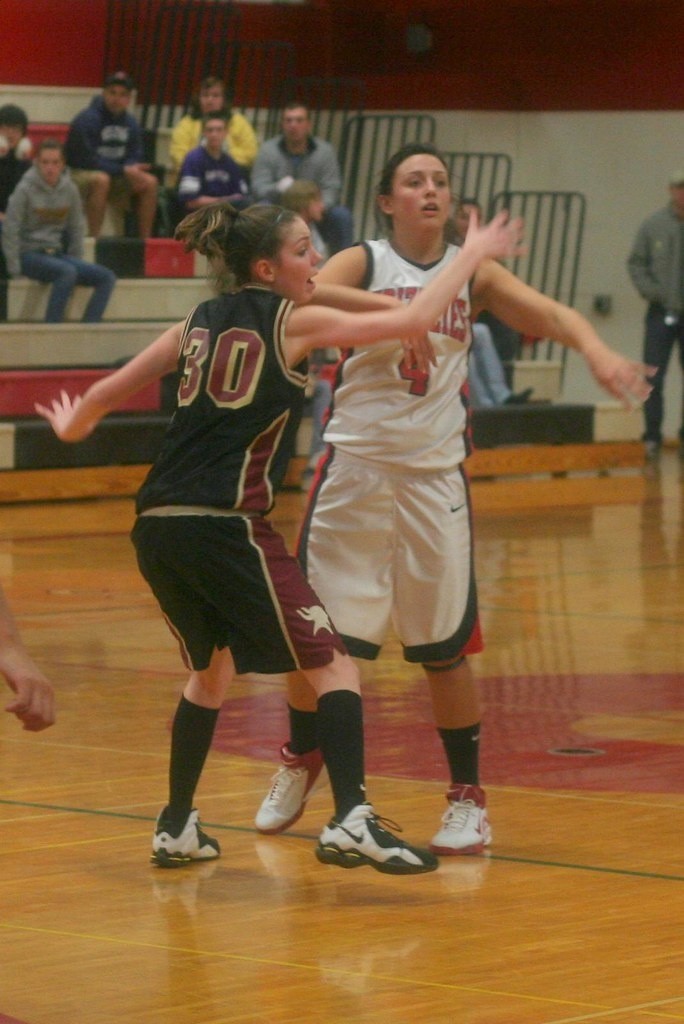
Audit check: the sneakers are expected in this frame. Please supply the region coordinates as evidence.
[150,806,221,866]
[315,802,439,874]
[429,784,492,855]
[255,744,324,834]
[255,836,319,904]
[438,856,492,889]
[148,865,214,916]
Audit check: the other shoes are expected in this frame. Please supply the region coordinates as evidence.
[504,389,532,404]
[645,442,659,460]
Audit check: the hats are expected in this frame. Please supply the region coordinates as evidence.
[103,71,134,91]
[668,171,684,186]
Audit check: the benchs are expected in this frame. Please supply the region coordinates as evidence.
[0,122,648,486]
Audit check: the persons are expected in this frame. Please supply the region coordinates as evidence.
[0,71,354,322]
[256,144,658,856]
[627,171,684,459]
[454,199,535,407]
[34,202,528,876]
